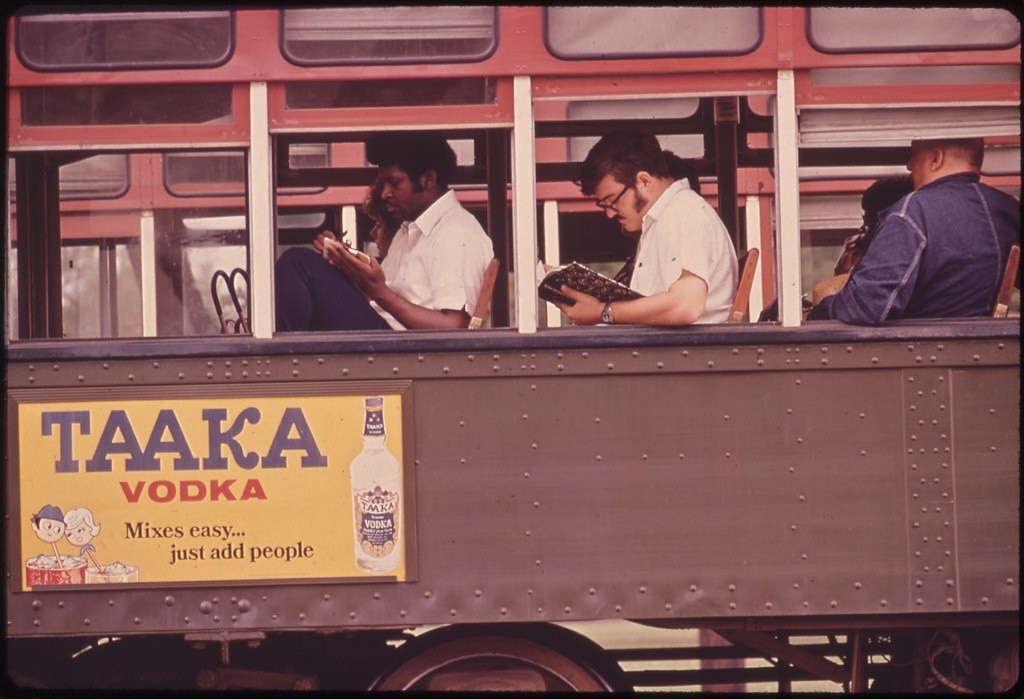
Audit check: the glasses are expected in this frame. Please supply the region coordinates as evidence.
[595,181,634,215]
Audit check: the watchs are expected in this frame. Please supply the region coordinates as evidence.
[601,302,614,325]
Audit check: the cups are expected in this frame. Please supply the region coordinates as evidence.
[27,556,86,587]
[86,565,139,582]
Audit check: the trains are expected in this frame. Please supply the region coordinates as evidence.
[7,4,1022,692]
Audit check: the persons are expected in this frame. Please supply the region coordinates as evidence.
[552,124,740,324]
[804,137,1023,327]
[273,129,494,332]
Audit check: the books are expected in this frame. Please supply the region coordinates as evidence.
[538,258,647,308]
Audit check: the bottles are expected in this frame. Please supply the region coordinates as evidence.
[350,397,402,574]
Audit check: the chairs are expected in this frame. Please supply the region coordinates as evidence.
[992,245,1020,318]
[468,259,501,329]
[729,248,760,323]
